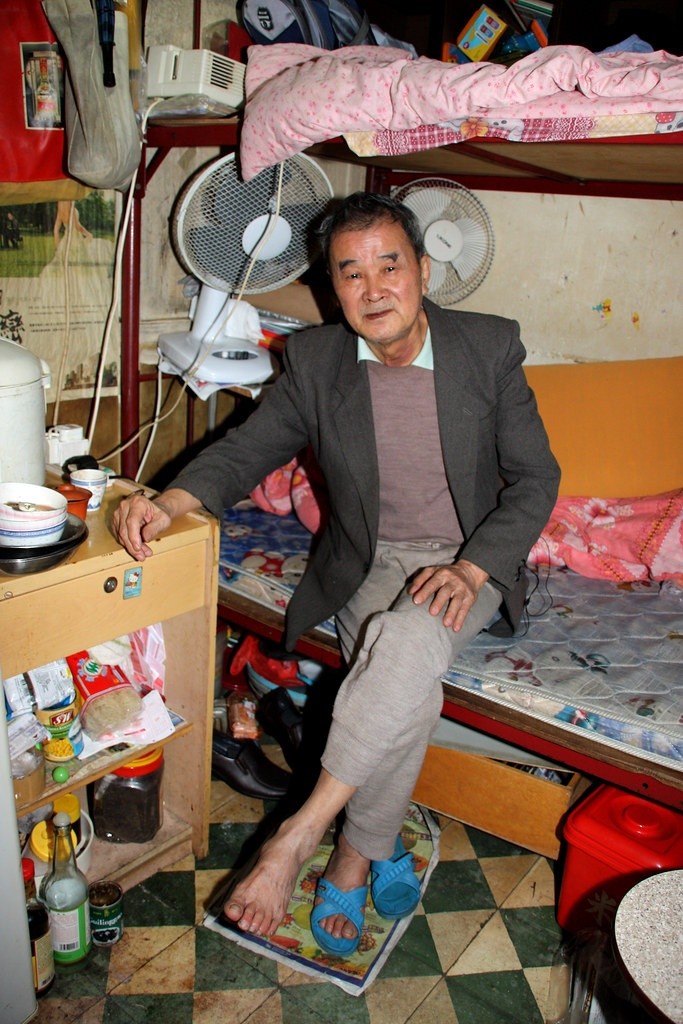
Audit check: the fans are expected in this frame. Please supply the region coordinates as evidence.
[389,177,494,306]
[157,149,335,384]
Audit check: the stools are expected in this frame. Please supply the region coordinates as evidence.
[570,870,683,1024]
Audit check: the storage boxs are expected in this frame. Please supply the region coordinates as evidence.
[410,744,683,947]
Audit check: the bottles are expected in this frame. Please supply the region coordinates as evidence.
[22,858,54,998]
[39,812,92,975]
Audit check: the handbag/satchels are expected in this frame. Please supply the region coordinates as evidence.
[40,0,141,194]
[234,0,420,61]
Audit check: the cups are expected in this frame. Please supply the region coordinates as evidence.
[71,469,107,511]
[55,483,92,521]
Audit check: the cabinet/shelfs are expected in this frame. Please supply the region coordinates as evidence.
[0,464,219,894]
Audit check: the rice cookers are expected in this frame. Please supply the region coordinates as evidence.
[0,336,50,487]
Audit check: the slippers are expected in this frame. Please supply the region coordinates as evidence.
[310,877,370,958]
[370,834,422,921]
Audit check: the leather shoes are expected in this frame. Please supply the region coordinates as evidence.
[212,728,301,800]
[255,687,320,774]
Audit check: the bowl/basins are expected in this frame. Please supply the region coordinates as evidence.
[0,483,90,574]
[23,808,93,892]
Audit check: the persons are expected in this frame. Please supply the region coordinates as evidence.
[108,193,561,958]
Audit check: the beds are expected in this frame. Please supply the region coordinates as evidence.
[120,97,683,813]
